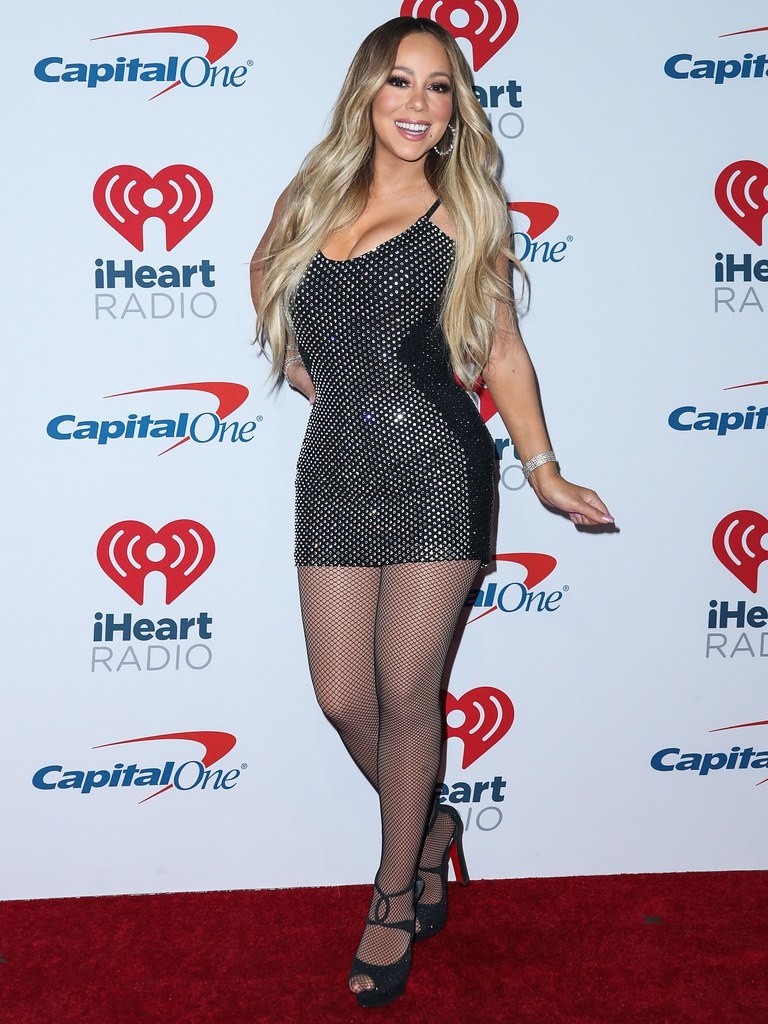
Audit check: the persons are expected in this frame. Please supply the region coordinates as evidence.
[249,18,615,1008]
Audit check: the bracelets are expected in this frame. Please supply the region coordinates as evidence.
[282,344,304,388]
[522,449,556,480]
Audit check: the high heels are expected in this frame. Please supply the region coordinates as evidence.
[348,871,424,1007]
[414,804,469,942]
